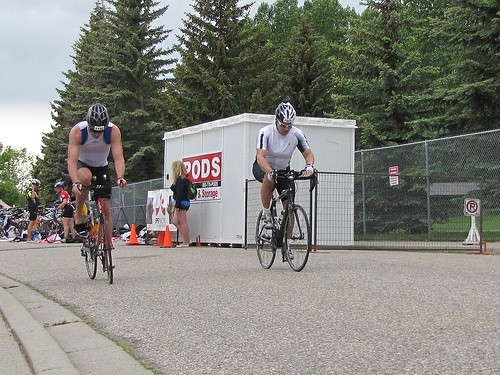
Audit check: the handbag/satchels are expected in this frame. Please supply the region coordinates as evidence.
[188,185,196,200]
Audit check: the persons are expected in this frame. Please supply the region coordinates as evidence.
[252,102,315,262]
[27,178,42,242]
[67,103,127,250]
[170,161,192,248]
[54,182,77,243]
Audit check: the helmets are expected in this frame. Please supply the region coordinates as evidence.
[86,103,109,129]
[275,102,296,123]
[29,178,41,186]
[54,182,62,187]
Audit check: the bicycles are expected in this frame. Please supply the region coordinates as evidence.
[0,204,84,243]
[255,169,320,272]
[75,175,124,284]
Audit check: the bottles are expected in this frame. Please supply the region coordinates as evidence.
[273,214,278,227]
[278,210,285,225]
[87,218,92,238]
[93,219,98,236]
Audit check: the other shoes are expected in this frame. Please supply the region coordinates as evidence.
[73,221,88,238]
[27,240,35,243]
[282,251,294,262]
[177,240,192,248]
[61,239,66,243]
[262,215,273,229]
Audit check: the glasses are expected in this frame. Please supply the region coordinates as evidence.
[277,120,293,128]
[90,127,103,133]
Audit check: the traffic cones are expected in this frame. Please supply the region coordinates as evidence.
[154,231,164,246]
[125,223,141,245]
[161,224,176,248]
